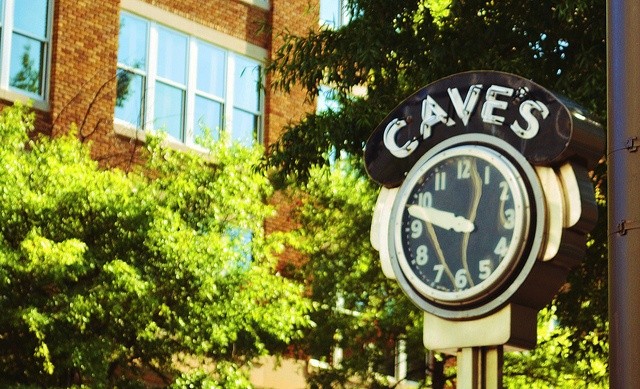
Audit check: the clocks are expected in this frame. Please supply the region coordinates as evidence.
[388,131,550,322]
